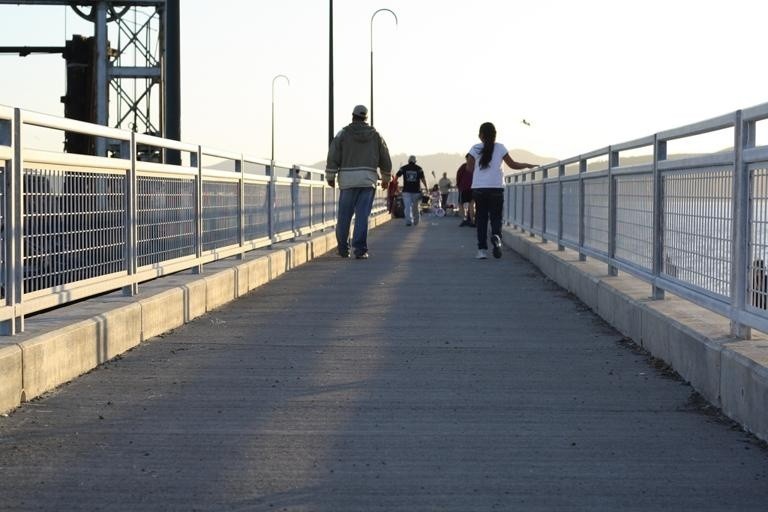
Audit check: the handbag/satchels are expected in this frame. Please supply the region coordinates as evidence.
[392,198,404,218]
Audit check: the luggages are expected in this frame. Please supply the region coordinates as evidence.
[436,207,445,216]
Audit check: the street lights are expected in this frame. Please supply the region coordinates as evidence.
[370,8,398,126]
[271,74,292,160]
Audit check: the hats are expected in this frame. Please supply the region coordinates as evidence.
[409,156,416,163]
[352,105,367,118]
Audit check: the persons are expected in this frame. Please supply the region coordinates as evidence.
[429,184,440,209]
[439,173,450,208]
[393,156,427,226]
[750,257,767,310]
[325,105,392,259]
[456,153,475,227]
[469,122,539,259]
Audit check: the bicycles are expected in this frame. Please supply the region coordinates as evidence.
[429,198,445,218]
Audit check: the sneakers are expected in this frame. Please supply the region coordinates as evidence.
[335,248,368,259]
[490,233,502,258]
[474,249,488,259]
